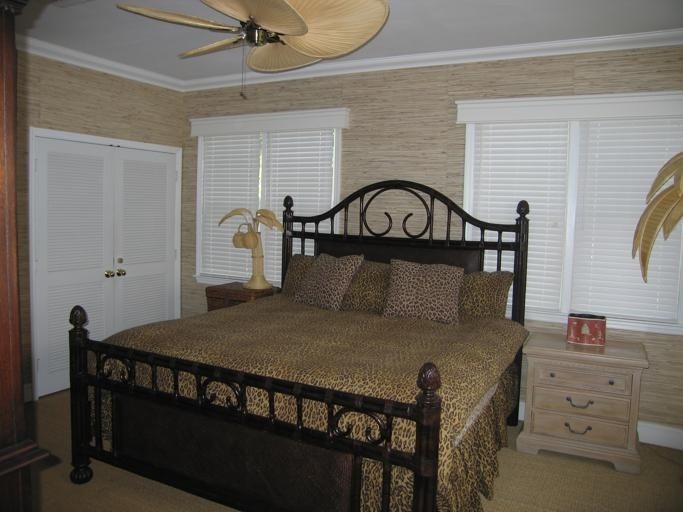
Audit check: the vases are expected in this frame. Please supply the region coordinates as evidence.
[243,233,274,291]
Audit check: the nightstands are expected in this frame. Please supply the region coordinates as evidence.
[514,331,650,477]
[203,280,274,310]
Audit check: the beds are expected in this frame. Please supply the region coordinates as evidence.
[67,175,533,511]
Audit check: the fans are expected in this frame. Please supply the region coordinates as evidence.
[116,1,391,74]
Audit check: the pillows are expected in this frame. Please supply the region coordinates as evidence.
[280,249,518,326]
